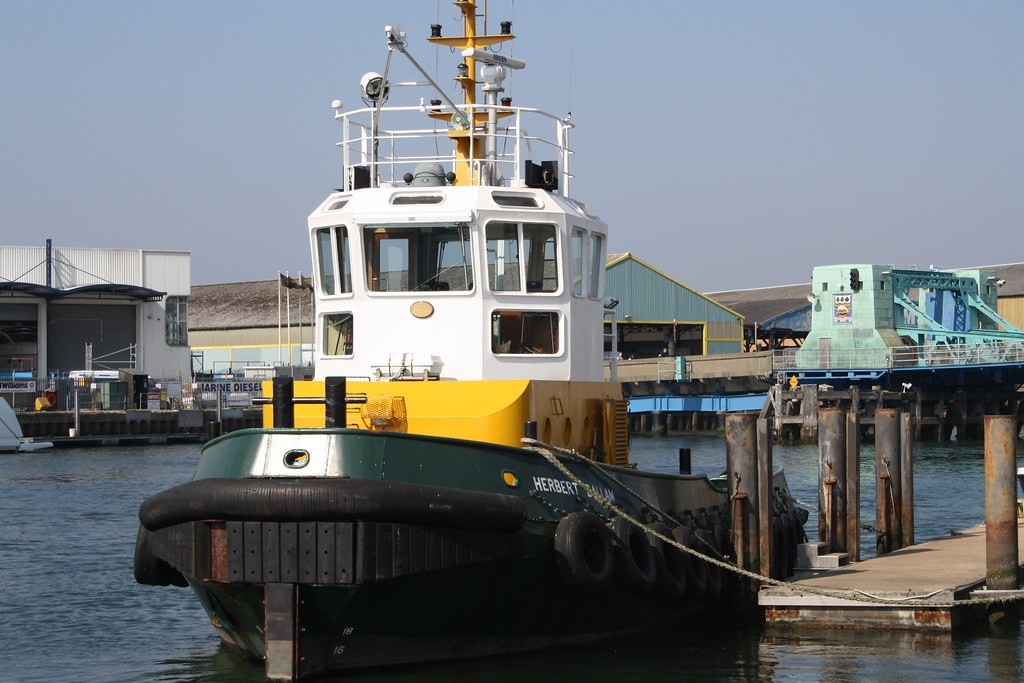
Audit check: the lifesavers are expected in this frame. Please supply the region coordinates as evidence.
[46,394,57,406]
[552,509,802,604]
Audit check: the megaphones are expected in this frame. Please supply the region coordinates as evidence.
[806,293,820,302]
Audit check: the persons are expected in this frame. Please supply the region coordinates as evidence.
[658,347,669,357]
[617,352,634,361]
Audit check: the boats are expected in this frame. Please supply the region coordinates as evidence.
[0,397,55,452]
[130,0,814,682]
